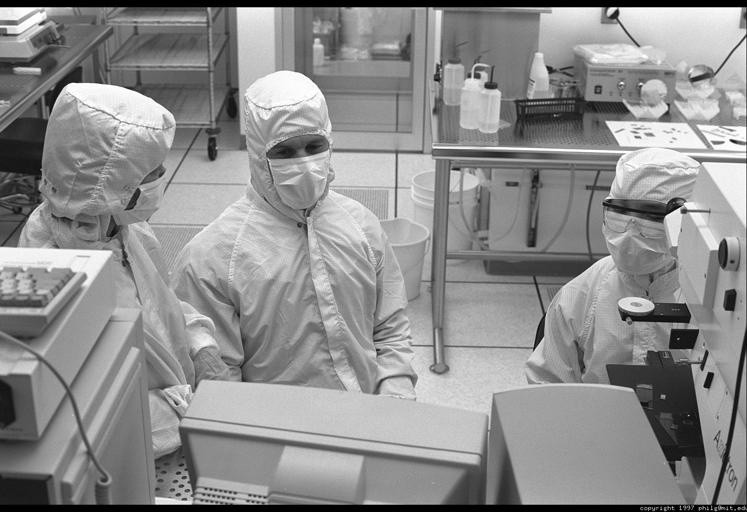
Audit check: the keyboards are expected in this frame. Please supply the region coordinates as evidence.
[0,267,86,338]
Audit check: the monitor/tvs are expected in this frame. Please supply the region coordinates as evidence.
[176,378,490,505]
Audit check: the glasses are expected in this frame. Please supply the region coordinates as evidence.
[602,200,666,239]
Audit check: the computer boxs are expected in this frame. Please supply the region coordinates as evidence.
[0,308,154,505]
[492,382,676,504]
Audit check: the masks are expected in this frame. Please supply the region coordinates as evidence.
[602,211,675,274]
[113,172,165,226]
[266,149,330,210]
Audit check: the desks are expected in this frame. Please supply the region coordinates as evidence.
[426,90,745,376]
[1,24,113,245]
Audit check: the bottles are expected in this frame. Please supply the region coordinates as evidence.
[444,57,501,134]
[313,38,324,66]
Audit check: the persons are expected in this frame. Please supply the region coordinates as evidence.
[169,70,418,406]
[19,82,233,462]
[525,147,700,385]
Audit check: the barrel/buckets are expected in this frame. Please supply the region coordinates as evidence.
[378,217,431,302]
[410,169,479,265]
